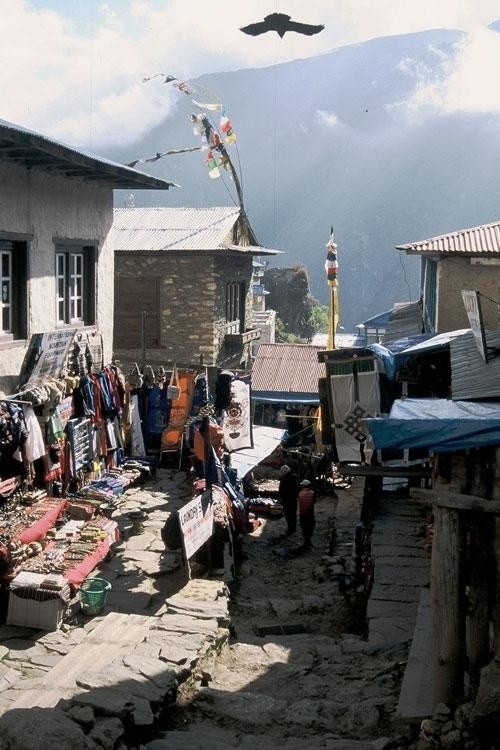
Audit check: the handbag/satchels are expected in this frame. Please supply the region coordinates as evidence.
[128,362,142,388]
[167,385,180,399]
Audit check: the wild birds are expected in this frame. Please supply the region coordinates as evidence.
[238,12,328,40]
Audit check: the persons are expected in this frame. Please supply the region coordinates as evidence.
[298,479,316,547]
[277,464,304,536]
[0,539,18,628]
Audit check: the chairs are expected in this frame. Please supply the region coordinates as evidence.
[159,432,183,471]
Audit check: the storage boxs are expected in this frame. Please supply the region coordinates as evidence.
[6,571,68,631]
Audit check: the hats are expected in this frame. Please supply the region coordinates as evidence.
[300,479,311,485]
